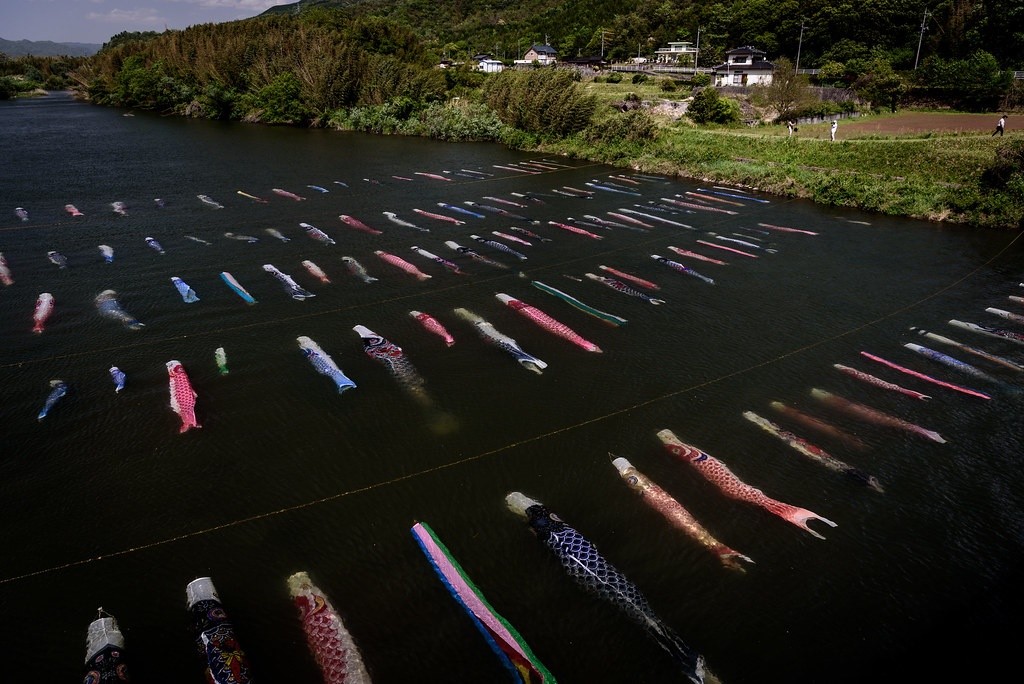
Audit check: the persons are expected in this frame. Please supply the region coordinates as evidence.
[830,120,837,141]
[992,115,1008,137]
[788,118,796,137]
